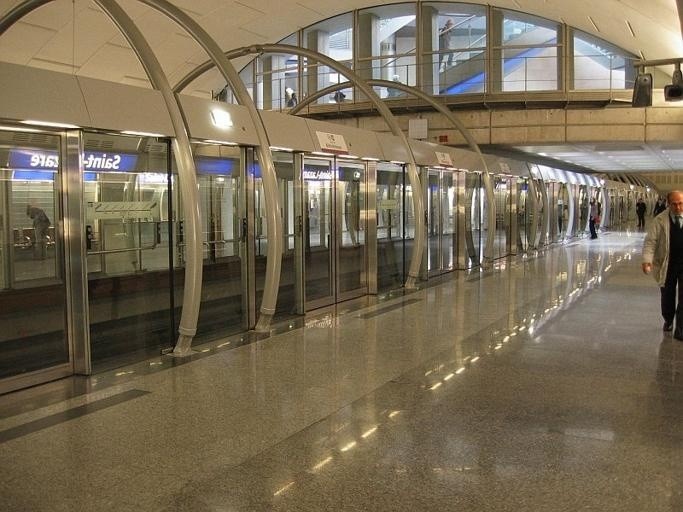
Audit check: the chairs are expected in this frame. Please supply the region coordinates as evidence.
[12,227,99,256]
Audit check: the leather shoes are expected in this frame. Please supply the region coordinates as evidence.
[663,321,673,331]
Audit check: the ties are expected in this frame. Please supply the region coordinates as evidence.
[674,215,681,227]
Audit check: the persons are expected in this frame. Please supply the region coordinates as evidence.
[28,204,51,260]
[589,191,666,238]
[642,190,683,342]
[334,92,346,103]
[439,19,454,70]
[285,86,294,101]
[288,93,297,107]
[387,75,402,98]
